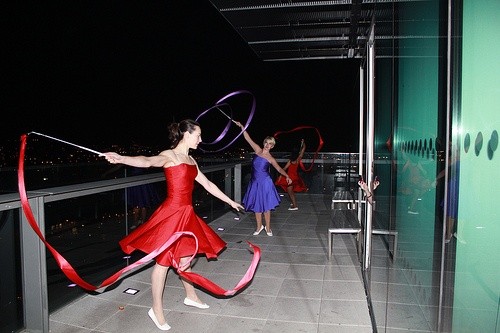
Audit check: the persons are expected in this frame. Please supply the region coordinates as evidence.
[431,149,460,243]
[402,155,427,215]
[98,119,245,331]
[102,149,162,229]
[278,139,306,210]
[236,122,292,237]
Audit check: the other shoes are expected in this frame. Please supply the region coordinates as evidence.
[265,226,272,236]
[288,207,298,210]
[148,308,171,331]
[184,298,210,308]
[253,225,264,235]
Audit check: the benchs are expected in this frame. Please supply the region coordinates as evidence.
[328,209,361,261]
[371,211,399,265]
[332,187,353,209]
